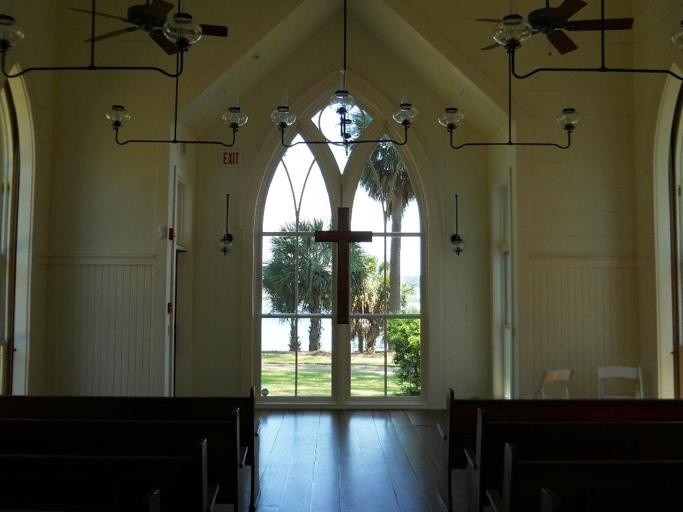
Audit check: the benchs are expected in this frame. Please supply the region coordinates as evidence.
[436,387,683,512]
[0,387,262,511]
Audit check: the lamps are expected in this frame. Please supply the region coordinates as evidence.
[447,193,464,255]
[216,194,233,255]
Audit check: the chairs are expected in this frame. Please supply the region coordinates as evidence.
[536,365,643,399]
